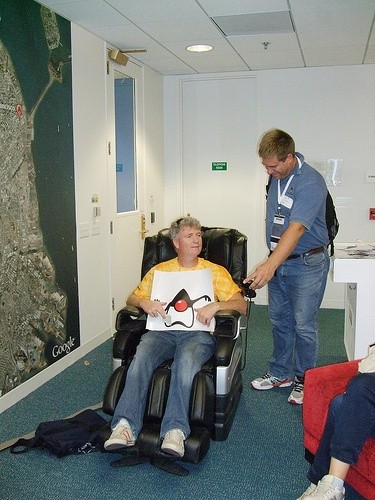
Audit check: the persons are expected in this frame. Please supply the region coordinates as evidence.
[243,129,330,405]
[104,217,248,458]
[296,343,375,500]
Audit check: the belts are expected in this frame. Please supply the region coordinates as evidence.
[270,246,324,260]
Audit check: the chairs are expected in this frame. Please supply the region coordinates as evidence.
[100,226,259,475]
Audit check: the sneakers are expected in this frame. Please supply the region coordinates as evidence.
[161,428,186,458]
[288,376,304,404]
[250,372,294,390]
[297,475,345,500]
[104,418,135,450]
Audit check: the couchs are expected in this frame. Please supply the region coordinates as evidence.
[303,360,375,500]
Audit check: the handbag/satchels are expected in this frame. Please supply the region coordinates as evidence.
[10,409,108,458]
[266,174,339,256]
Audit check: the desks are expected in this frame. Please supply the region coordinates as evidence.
[329,242,375,362]
